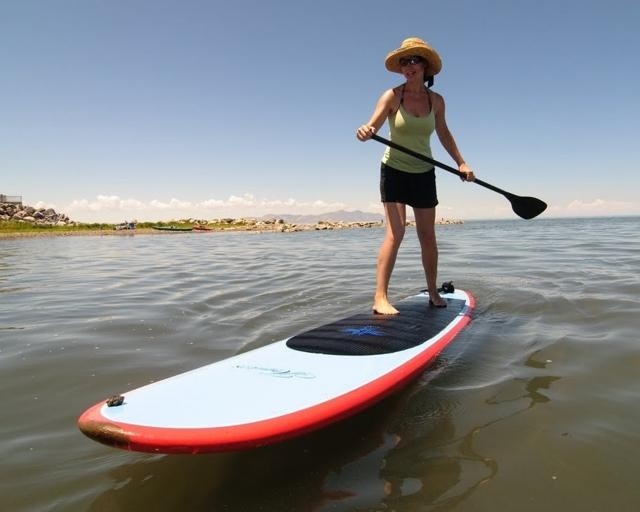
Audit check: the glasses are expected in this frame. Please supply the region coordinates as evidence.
[398,54,425,66]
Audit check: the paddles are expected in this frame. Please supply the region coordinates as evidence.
[369,129,547,220]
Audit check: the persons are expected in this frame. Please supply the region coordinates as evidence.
[356,38,476,315]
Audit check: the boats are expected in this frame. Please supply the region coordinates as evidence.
[152,225,214,231]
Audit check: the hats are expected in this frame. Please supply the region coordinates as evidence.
[384,36,442,79]
